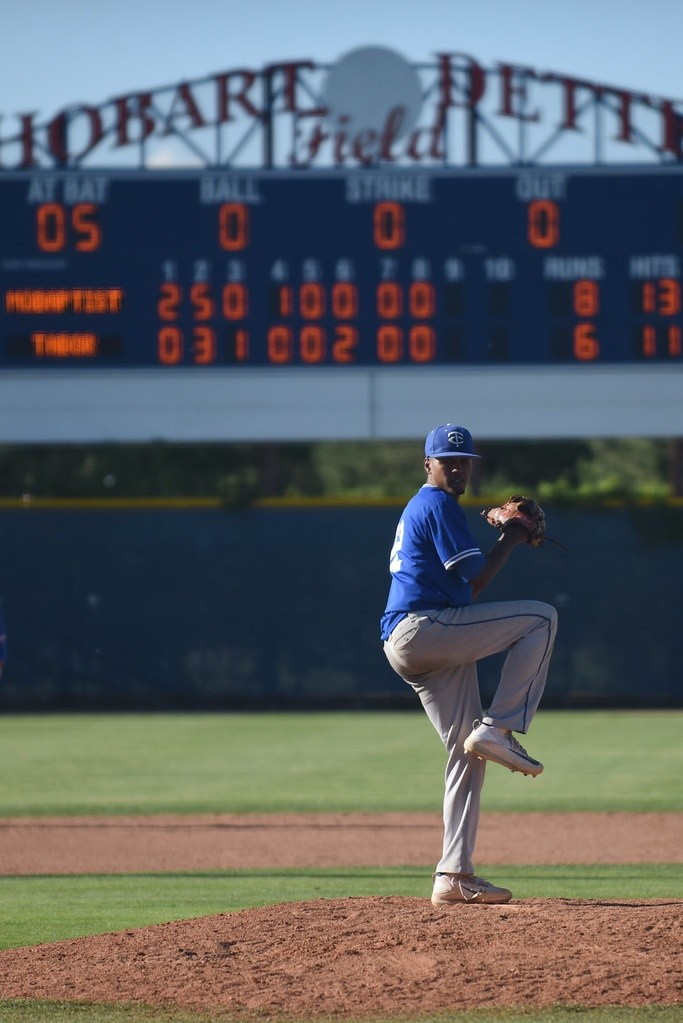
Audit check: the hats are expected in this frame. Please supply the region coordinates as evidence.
[425,423,482,458]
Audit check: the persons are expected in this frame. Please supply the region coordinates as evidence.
[380,423,558,905]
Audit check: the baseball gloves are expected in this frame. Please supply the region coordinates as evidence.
[479,494,568,551]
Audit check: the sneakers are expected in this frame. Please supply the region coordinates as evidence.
[464,719,543,777]
[431,872,512,905]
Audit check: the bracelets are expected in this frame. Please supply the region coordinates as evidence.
[487,532,519,554]
